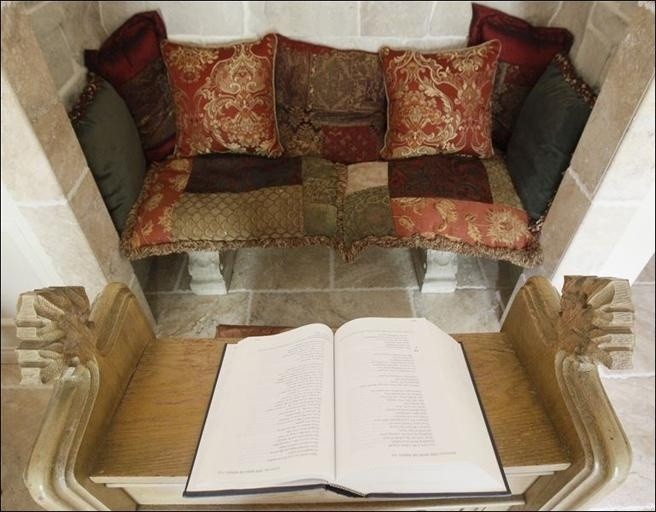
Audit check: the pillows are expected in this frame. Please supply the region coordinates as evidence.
[378,40,502,160]
[504,55,597,230]
[161,34,285,158]
[274,34,386,132]
[467,7,574,142]
[83,11,177,162]
[71,72,147,231]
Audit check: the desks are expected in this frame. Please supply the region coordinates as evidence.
[15,273,634,512]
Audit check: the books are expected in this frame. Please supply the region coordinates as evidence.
[178,316,512,501]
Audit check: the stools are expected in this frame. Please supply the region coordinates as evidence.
[214,324,294,338]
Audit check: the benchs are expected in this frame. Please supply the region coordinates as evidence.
[118,153,543,293]
